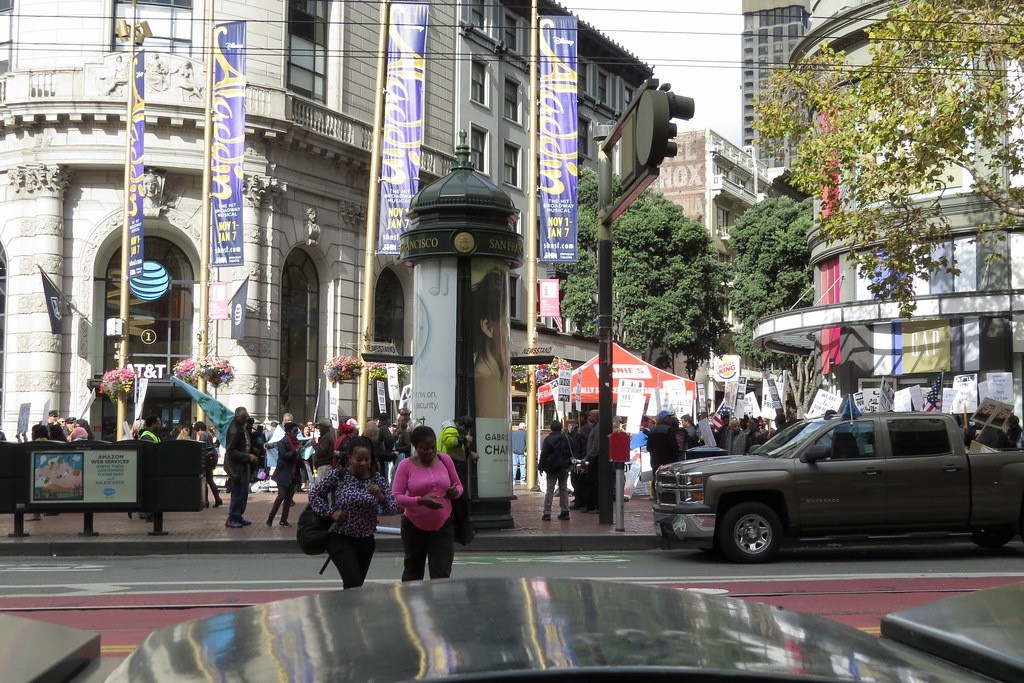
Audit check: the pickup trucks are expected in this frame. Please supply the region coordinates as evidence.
[651,408,1024,562]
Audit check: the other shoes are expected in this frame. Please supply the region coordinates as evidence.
[214,498,223,507]
[558,511,570,519]
[570,505,599,514]
[542,514,551,520]
[225,517,241,527]
[278,520,291,526]
[128,512,132,519]
[26,516,40,520]
[267,517,273,527]
[236,517,250,526]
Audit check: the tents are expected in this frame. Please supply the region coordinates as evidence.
[537,341,696,465]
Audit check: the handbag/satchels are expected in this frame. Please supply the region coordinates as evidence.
[569,458,576,472]
[296,503,335,555]
[452,494,474,544]
[207,447,217,467]
[257,468,268,480]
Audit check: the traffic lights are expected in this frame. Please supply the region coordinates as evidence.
[635,92,695,167]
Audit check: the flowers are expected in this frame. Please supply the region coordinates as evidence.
[511,365,533,386]
[323,355,364,386]
[172,358,198,389]
[534,357,573,387]
[193,355,238,392]
[99,367,146,405]
[365,362,411,383]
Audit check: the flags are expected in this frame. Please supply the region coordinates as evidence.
[230,275,248,339]
[41,271,63,334]
[171,375,235,446]
[921,372,944,412]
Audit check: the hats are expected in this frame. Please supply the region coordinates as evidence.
[658,410,675,420]
[49,409,58,417]
[551,420,563,431]
[720,411,731,419]
[348,418,359,429]
[398,407,411,415]
[461,416,474,429]
[316,419,331,427]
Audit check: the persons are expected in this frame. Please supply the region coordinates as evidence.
[393,425,464,582]
[143,172,159,198]
[308,436,399,590]
[470,266,510,418]
[0,406,1023,545]
[306,206,320,239]
